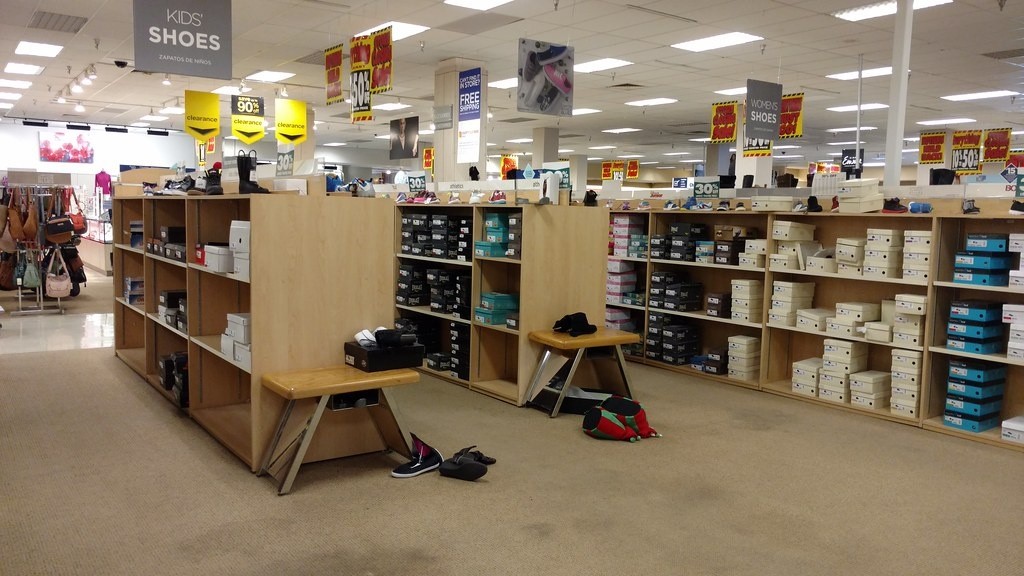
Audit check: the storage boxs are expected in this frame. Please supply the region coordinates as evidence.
[220,311,252,372]
[750,196,794,213]
[395,208,523,381]
[837,177,886,216]
[122,214,252,411]
[605,214,1024,444]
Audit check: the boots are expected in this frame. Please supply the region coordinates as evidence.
[237,156,272,195]
[582,190,598,207]
[203,168,224,196]
[929,168,956,186]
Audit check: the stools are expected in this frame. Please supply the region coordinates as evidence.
[522,325,641,419]
[254,364,417,497]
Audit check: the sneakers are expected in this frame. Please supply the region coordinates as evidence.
[635,200,652,210]
[489,188,506,205]
[452,444,496,466]
[334,177,376,198]
[468,188,484,204]
[381,190,441,205]
[391,453,439,479]
[831,195,839,213]
[961,197,981,215]
[621,199,631,211]
[907,199,935,216]
[715,200,730,211]
[661,199,680,211]
[734,201,746,212]
[881,197,908,215]
[604,199,613,209]
[447,190,462,205]
[327,174,345,193]
[689,200,714,211]
[680,196,696,212]
[439,456,488,484]
[411,443,444,466]
[807,194,823,214]
[1007,198,1024,217]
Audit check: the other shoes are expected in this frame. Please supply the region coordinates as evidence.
[715,172,815,189]
[581,405,642,444]
[522,42,575,113]
[598,394,663,440]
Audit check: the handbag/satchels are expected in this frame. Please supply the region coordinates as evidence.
[0,186,88,303]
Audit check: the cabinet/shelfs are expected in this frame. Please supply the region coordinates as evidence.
[603,208,1022,449]
[112,198,399,472]
[396,204,614,409]
